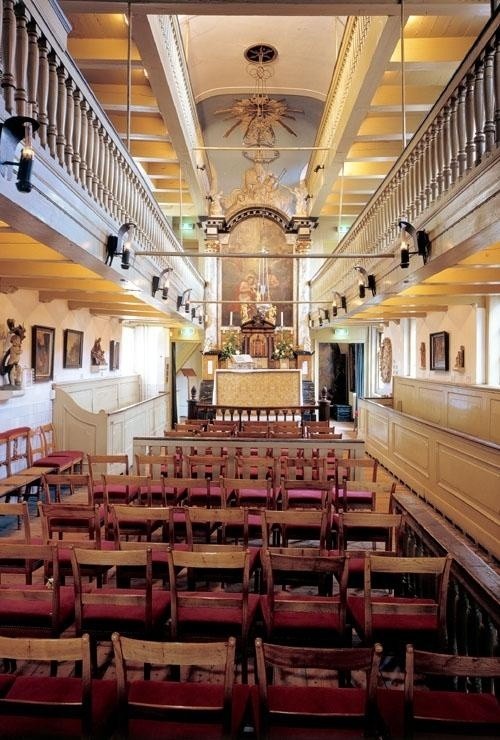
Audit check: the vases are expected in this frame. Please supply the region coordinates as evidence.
[279,357,290,369]
[220,358,231,369]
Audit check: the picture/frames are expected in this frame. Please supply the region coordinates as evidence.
[64,328,84,368]
[429,330,450,371]
[30,326,55,383]
[109,340,120,370]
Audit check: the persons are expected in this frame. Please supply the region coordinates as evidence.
[237,275,258,318]
[210,173,309,217]
[6,334,24,386]
[260,266,280,294]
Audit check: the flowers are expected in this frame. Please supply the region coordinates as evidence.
[217,333,239,362]
[271,331,297,359]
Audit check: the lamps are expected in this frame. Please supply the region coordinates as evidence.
[304,217,434,327]
[242,51,283,319]
